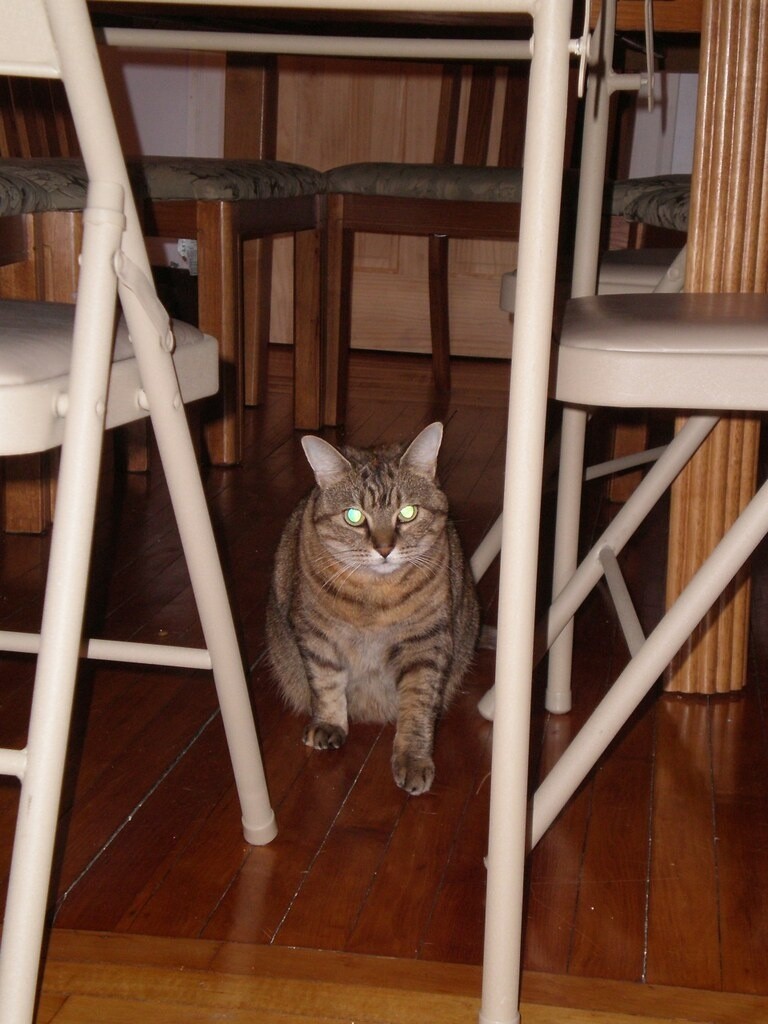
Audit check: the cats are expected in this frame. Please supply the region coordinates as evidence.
[264,421,482,798]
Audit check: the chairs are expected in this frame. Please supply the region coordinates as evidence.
[0,0,768,1024]
